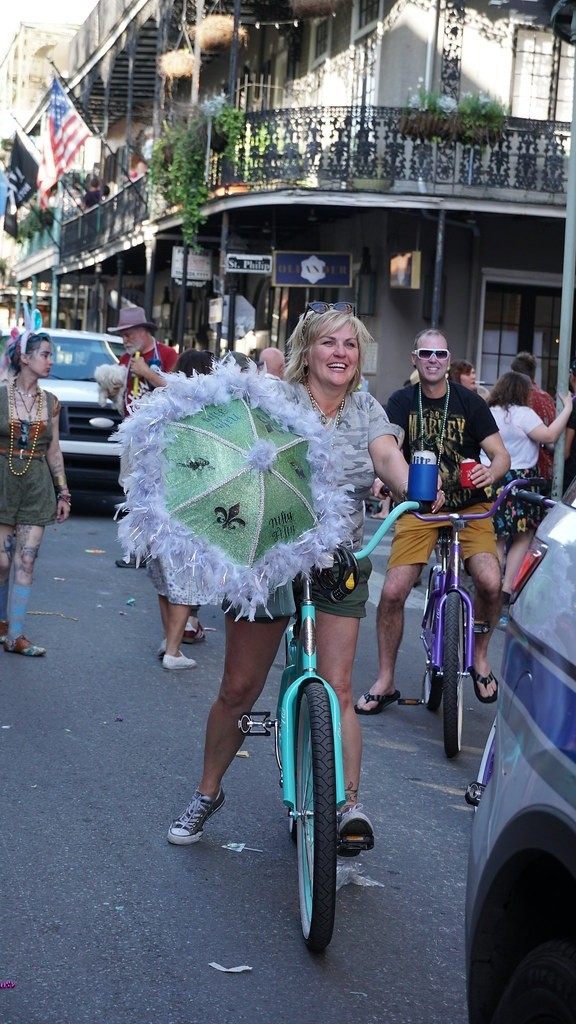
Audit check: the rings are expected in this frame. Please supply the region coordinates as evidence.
[442,491,445,496]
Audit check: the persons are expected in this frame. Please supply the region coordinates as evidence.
[356,328,511,714]
[82,178,99,210]
[168,311,445,858]
[108,307,179,568]
[414,359,490,588]
[0,301,71,655]
[122,126,153,183]
[490,352,576,613]
[370,495,390,518]
[148,347,287,669]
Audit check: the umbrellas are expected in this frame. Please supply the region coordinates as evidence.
[112,352,355,614]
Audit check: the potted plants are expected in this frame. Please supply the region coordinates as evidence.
[15,207,52,242]
[145,101,245,254]
[402,86,504,144]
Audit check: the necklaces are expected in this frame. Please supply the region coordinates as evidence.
[305,380,345,448]
[9,379,42,475]
[419,377,450,466]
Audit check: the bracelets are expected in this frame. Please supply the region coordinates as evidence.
[55,475,71,506]
[398,481,408,497]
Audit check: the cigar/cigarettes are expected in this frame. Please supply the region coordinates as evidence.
[474,381,485,384]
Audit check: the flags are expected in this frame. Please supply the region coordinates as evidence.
[40,79,92,210]
[0,132,41,239]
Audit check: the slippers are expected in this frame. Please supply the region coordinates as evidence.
[471,668,498,703]
[354,689,399,715]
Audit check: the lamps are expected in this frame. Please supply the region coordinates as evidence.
[354,242,377,318]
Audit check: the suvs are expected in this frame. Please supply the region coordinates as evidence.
[26,328,127,500]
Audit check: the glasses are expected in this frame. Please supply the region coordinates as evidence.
[303,302,355,318]
[18,419,30,449]
[414,348,451,360]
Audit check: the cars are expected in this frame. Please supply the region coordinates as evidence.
[466,477,576,1024]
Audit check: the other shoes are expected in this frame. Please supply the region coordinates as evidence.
[0,621,9,642]
[4,635,46,656]
[157,639,167,655]
[163,650,196,669]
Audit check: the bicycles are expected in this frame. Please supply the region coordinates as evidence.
[238,451,441,953]
[380,473,549,762]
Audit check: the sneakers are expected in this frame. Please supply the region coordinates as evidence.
[336,803,373,857]
[167,786,226,845]
[115,554,146,568]
[181,622,205,643]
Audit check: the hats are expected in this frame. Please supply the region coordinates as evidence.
[219,351,264,371]
[107,307,157,333]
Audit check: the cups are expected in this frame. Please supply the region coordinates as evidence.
[460,462,478,489]
[408,464,438,502]
